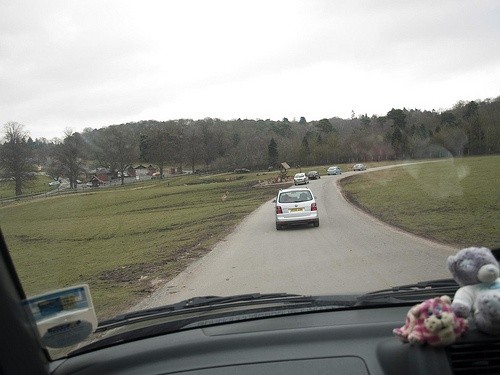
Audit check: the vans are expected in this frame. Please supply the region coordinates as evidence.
[293,172,308,185]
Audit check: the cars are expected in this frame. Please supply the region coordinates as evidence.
[49,181,60,186]
[327,167,342,175]
[353,163,367,172]
[308,170,320,179]
[86,181,99,186]
[76,179,83,183]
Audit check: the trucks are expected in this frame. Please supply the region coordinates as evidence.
[235,168,249,173]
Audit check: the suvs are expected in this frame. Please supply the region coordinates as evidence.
[273,187,319,229]
